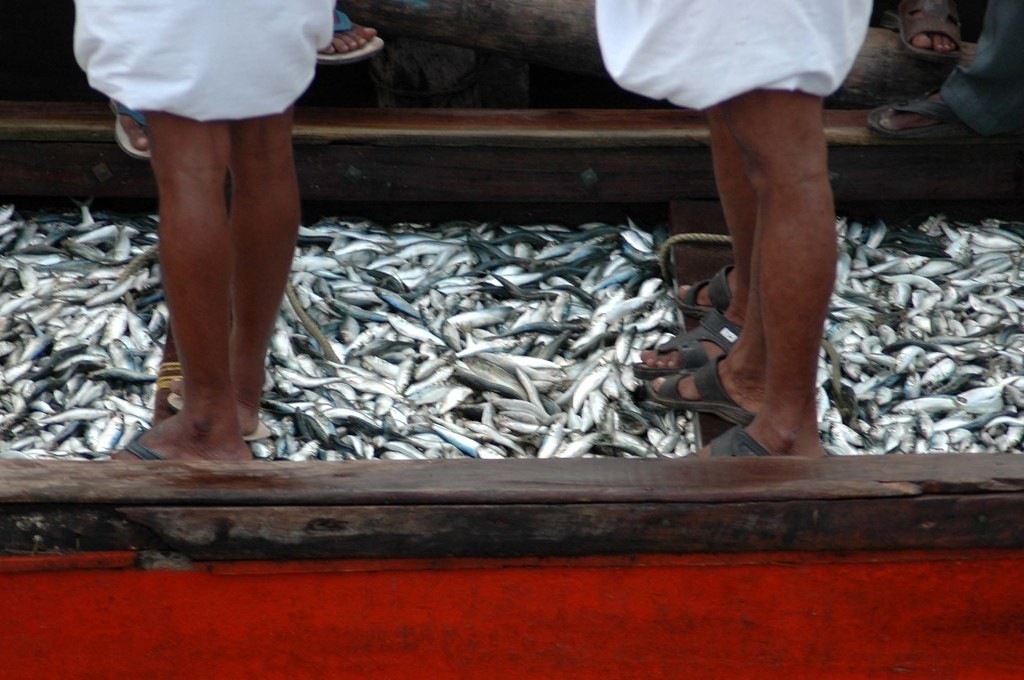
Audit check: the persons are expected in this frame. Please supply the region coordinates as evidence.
[590,0,1024,460]
[109,7,384,155]
[73,0,336,462]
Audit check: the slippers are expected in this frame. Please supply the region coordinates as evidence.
[108,98,151,160]
[633,308,743,380]
[681,424,774,456]
[166,392,272,441]
[867,84,981,139]
[877,0,962,62]
[675,264,733,319]
[122,429,164,460]
[317,9,385,67]
[642,354,756,424]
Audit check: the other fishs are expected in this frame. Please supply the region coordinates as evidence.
[1,202,700,459]
[814,213,1024,454]
